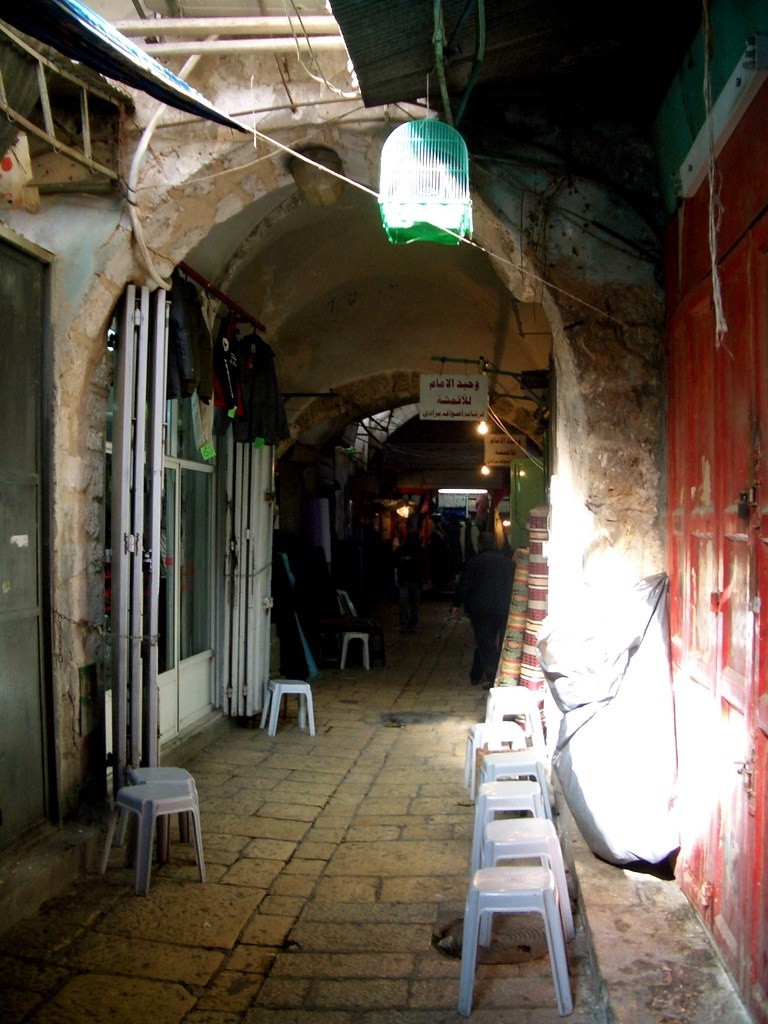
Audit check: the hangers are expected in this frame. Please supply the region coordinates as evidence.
[181,267,257,340]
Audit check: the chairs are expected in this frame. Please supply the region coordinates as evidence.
[336,589,385,668]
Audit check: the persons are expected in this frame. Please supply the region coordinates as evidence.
[393,535,432,632]
[453,531,515,689]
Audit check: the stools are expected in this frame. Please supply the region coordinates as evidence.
[456,684,581,1017]
[339,631,370,670]
[258,679,316,737]
[102,784,207,895]
[116,766,199,866]
